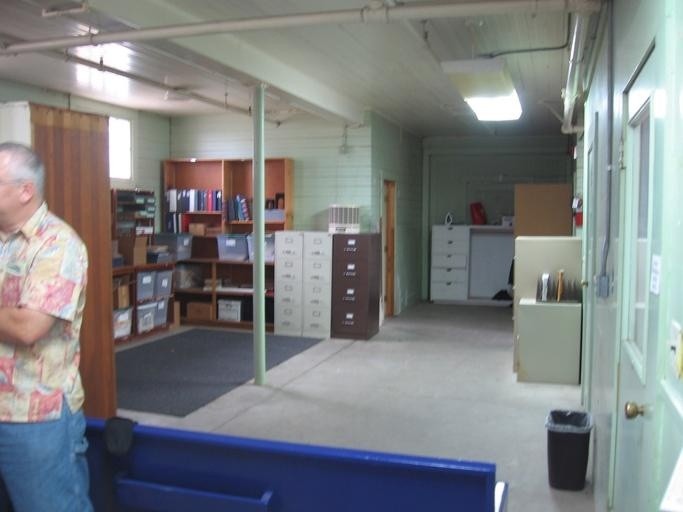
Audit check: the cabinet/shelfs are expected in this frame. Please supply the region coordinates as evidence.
[0,101,118,417]
[511,235,583,373]
[271,229,332,338]
[332,233,382,340]
[428,223,512,308]
[110,189,174,343]
[517,298,582,386]
[514,183,572,234]
[160,157,294,332]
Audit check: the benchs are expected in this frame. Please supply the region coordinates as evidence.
[77,419,508,512]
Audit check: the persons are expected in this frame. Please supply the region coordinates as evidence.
[0,141,95,512]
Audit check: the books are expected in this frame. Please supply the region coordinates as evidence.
[226,194,252,221]
[163,186,222,235]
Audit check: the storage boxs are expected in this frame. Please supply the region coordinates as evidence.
[111,229,275,341]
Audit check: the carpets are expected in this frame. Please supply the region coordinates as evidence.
[116,327,324,418]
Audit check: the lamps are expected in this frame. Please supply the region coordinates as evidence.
[439,48,521,121]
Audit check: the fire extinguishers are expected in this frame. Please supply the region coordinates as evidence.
[575,198,582,226]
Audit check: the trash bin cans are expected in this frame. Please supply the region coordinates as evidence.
[545,409,593,490]
